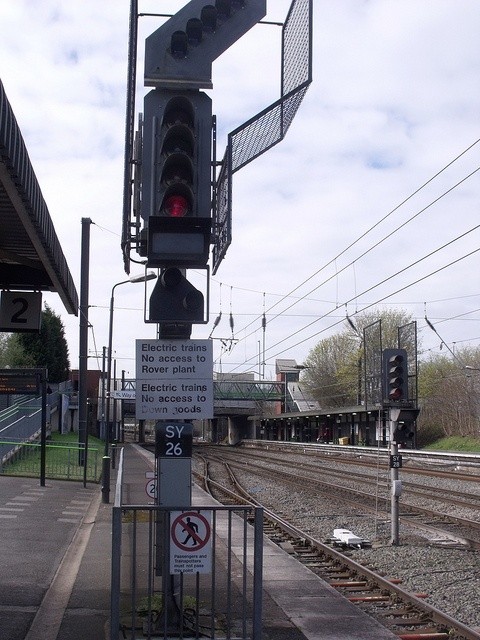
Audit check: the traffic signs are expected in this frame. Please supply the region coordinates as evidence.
[155,421,192,458]
[145,479,158,500]
[135,338,215,420]
[391,454,402,467]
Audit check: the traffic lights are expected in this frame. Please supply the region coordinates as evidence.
[392,419,414,444]
[382,348,409,403]
[144,0,267,84]
[150,264,205,319]
[144,88,213,218]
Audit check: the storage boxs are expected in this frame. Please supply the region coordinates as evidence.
[339,437,348,445]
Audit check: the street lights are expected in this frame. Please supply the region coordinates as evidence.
[465,365,480,373]
[104,270,157,447]
